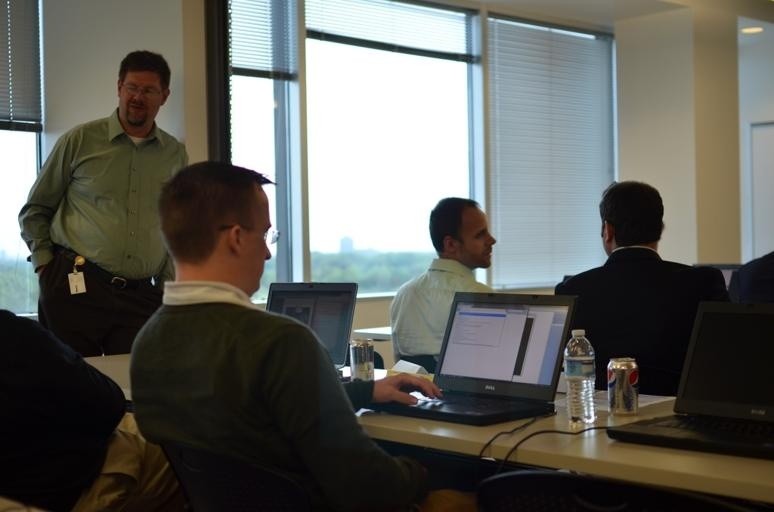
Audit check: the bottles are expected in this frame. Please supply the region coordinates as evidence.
[562,329,597,429]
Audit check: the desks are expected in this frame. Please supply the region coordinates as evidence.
[84,351,774,512]
[351,321,395,344]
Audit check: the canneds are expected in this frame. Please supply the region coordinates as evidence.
[606,357,638,415]
[350,338,374,382]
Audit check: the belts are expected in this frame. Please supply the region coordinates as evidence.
[53,246,160,290]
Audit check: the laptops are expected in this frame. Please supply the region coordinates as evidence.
[374,291,578,425]
[607,302,774,461]
[265,283,358,368]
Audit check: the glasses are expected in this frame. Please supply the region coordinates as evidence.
[124,81,160,99]
[219,224,279,244]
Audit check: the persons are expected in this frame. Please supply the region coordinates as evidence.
[16,49,189,357]
[129,161,477,512]
[727,250,773,304]
[0,309,185,510]
[387,195,497,376]
[553,180,727,398]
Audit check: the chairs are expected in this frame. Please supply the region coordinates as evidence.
[157,437,334,512]
